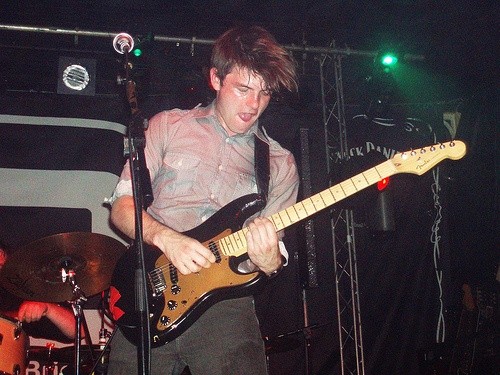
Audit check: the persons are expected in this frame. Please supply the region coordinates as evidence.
[447,261,474,315]
[107,25,300,375]
[0,241,86,346]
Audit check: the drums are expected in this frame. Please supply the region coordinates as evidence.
[0,313,29,375]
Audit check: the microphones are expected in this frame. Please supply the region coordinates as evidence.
[113,32,134,54]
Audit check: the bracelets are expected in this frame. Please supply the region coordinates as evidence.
[265,258,283,278]
[42,303,50,316]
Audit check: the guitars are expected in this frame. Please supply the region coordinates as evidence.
[107,140,466,347]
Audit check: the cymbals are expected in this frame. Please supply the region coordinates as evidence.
[0,231,126,304]
